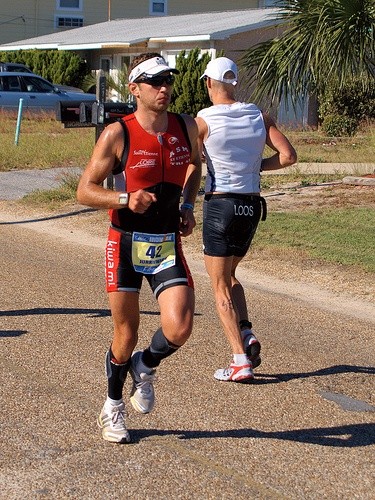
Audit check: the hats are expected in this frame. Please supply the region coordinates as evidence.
[129,56,180,83]
[198,56,238,86]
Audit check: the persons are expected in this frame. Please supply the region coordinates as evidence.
[193,56,298,380]
[77,53,203,443]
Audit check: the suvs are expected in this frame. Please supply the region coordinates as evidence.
[0,60,84,93]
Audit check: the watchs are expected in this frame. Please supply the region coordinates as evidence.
[119,192,129,207]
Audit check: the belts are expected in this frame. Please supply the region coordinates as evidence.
[204,193,267,221]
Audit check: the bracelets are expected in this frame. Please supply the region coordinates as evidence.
[181,203,194,211]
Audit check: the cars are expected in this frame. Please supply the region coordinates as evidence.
[0,72,96,113]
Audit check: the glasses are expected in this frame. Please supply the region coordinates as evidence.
[133,74,175,86]
[204,75,210,81]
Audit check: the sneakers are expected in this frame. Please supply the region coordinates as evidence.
[98,399,132,444]
[213,357,254,381]
[127,350,155,414]
[240,327,262,368]
